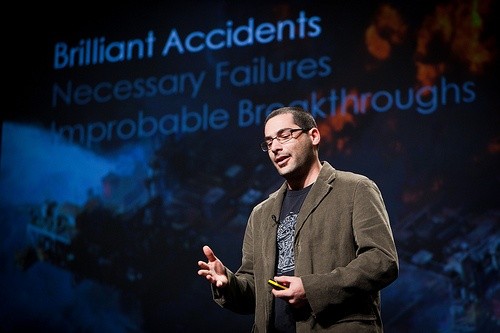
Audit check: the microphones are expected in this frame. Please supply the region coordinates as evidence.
[272,214,276,222]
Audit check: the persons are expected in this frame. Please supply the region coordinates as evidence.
[198,107,399,333]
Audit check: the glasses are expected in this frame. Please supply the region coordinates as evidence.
[261,129,311,152]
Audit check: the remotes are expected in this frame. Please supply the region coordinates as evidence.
[268,279,289,290]
